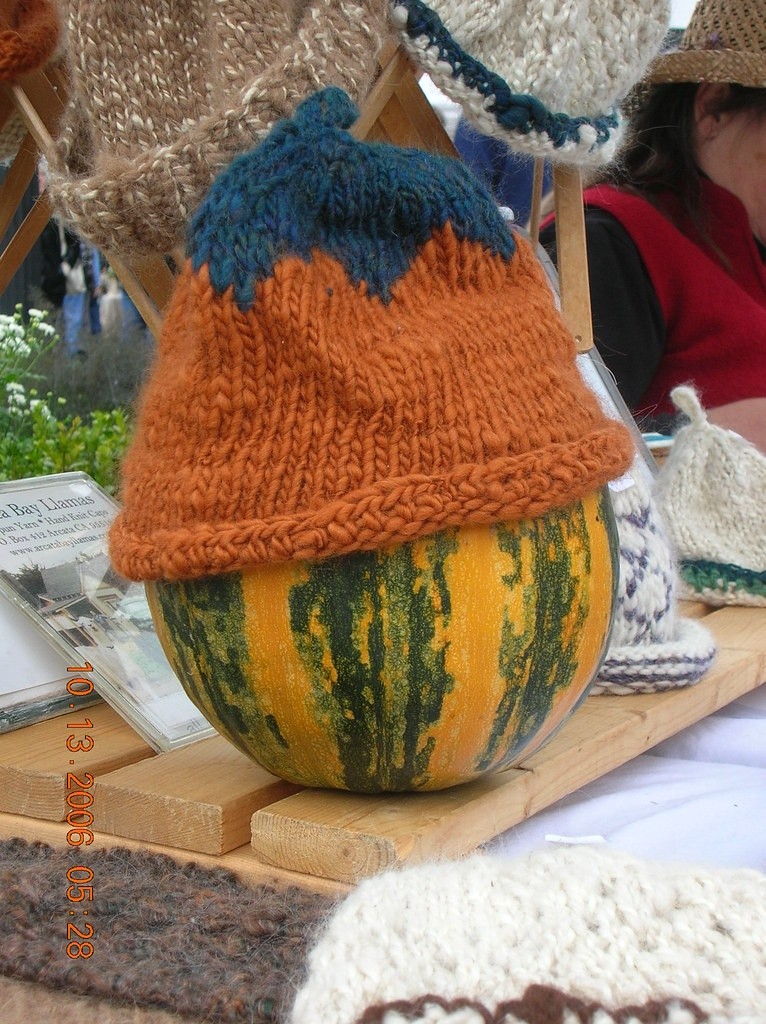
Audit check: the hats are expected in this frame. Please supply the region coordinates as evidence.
[654,383,765,606]
[110,86,634,580]
[39,0,384,249]
[2,0,60,75]
[389,0,672,166]
[589,460,715,696]
[650,1,765,90]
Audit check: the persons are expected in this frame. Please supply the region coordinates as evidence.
[532,0,766,454]
[59,245,101,359]
[455,116,552,231]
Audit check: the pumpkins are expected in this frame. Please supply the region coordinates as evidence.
[146,482,622,793]
